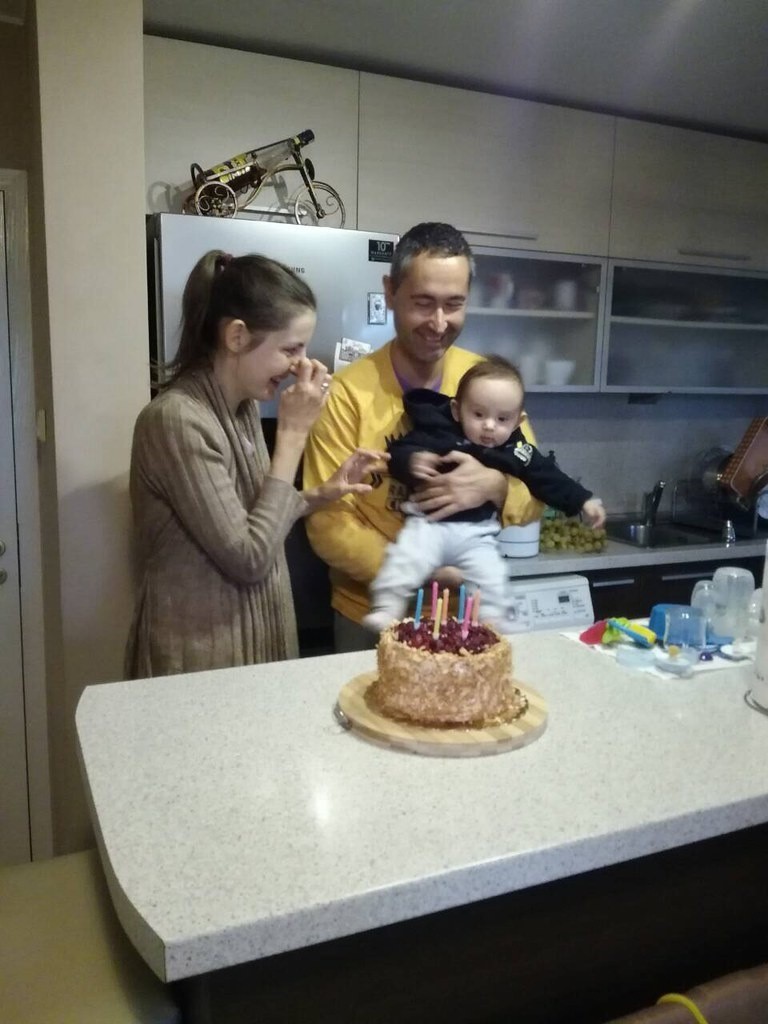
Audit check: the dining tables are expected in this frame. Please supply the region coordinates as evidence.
[74,628,768,1024]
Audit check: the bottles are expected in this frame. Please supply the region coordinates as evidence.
[197,130,315,193]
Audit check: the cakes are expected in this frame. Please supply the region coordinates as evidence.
[375,615,513,723]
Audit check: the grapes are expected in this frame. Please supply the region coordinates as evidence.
[539,518,609,554]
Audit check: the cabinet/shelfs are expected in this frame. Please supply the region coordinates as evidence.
[444,246,609,395]
[587,555,765,623]
[359,70,613,257]
[142,34,359,233]
[601,258,768,394]
[610,112,768,273]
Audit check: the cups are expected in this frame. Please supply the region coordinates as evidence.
[553,281,577,311]
[518,354,537,385]
[548,361,574,386]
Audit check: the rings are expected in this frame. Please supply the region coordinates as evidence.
[319,381,330,393]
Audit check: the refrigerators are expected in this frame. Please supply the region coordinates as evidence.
[147,212,400,491]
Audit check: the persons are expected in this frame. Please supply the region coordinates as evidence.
[300,221,545,654]
[360,352,606,637]
[120,250,391,681]
